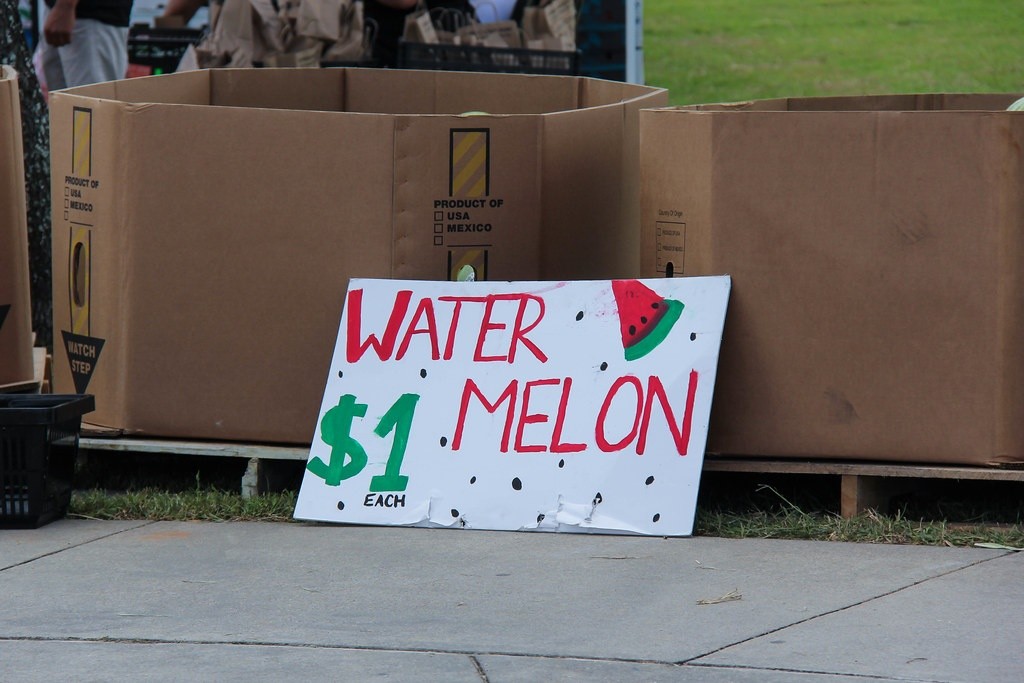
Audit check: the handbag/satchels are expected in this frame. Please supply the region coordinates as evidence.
[402,0,577,51]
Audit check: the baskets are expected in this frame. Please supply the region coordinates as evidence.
[397,37,581,75]
[0,393,96,530]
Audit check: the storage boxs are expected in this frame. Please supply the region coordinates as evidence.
[128,26,211,66]
[47,68,669,447]
[640,93,1024,469]
[0,393,96,529]
[0,64,37,393]
[401,41,582,75]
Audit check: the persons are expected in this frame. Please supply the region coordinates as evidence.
[161,1,203,27]
[32,0,135,105]
